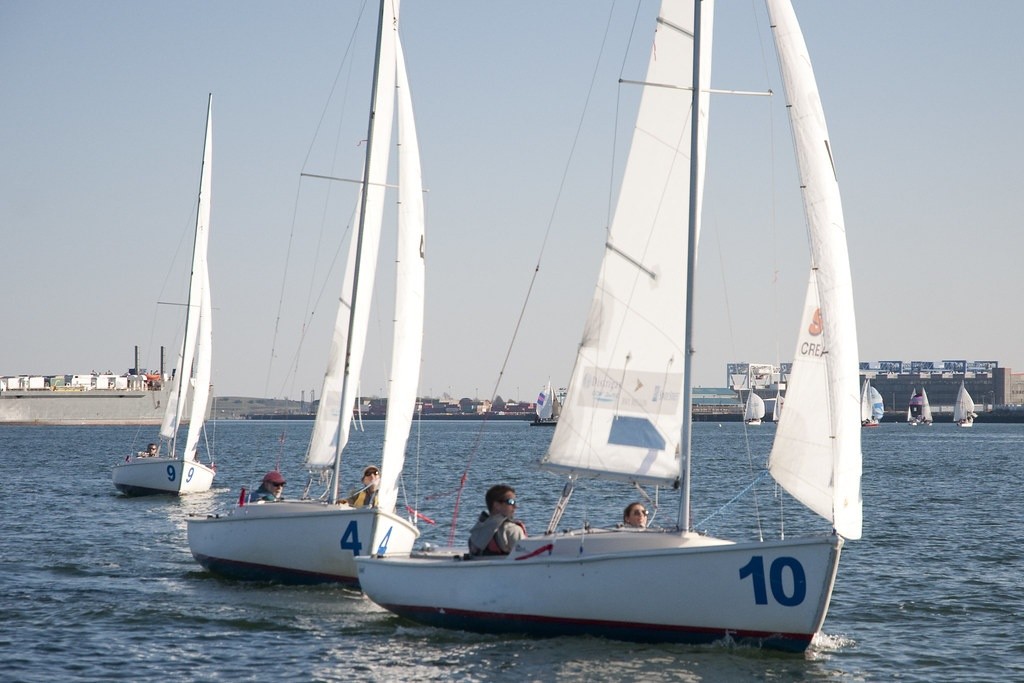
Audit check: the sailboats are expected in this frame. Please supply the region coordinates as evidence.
[743,379,978,426]
[112,93,215,493]
[184,1,864,650]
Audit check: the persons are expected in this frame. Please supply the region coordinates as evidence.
[140,443,156,458]
[624,502,649,527]
[911,417,927,423]
[469,485,527,555]
[246,471,286,502]
[336,465,380,507]
[959,417,971,424]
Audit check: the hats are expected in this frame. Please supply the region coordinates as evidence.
[263,471,287,484]
[361,466,379,480]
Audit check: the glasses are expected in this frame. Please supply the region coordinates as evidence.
[365,470,379,476]
[632,510,649,516]
[497,499,516,506]
[148,448,156,450]
[270,482,283,487]
